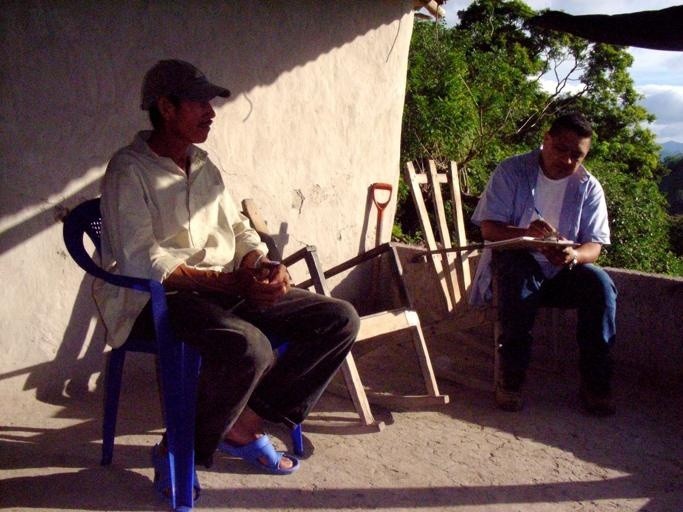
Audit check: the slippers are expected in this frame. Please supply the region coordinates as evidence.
[219,433,299,474]
[151,443,201,505]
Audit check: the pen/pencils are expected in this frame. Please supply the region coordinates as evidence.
[533,207,565,240]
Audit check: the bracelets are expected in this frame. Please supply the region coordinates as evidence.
[254,254,264,269]
[569,249,578,271]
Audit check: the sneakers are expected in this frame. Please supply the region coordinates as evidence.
[581,387,614,416]
[495,377,522,412]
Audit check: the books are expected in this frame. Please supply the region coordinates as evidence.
[484,235,582,250]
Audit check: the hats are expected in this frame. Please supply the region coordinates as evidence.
[141,60,230,110]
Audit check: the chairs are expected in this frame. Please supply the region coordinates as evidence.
[402,152,572,399]
[62,195,309,512]
[241,196,451,432]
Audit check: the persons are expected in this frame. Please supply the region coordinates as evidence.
[469,114,618,418]
[92,58,360,502]
